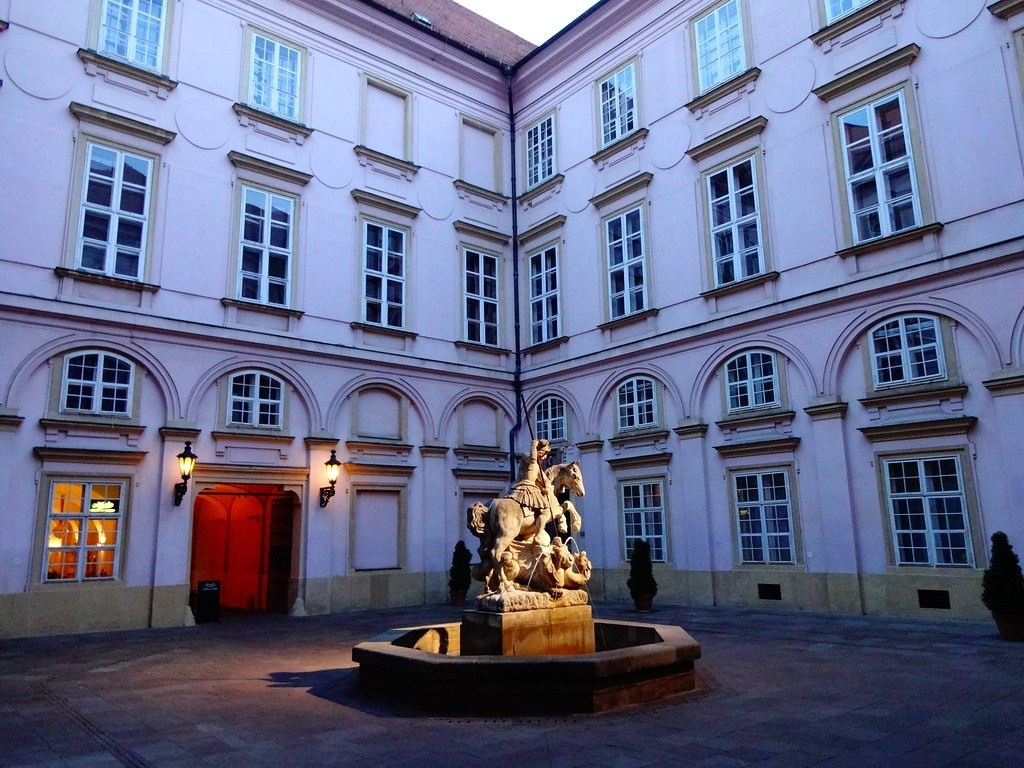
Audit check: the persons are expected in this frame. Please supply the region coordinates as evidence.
[501,439,552,546]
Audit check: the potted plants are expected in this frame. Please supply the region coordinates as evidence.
[980,530,1024,641]
[448,540,473,604]
[626,538,658,612]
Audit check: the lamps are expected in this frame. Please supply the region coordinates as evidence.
[320,449,342,508]
[174,440,198,506]
